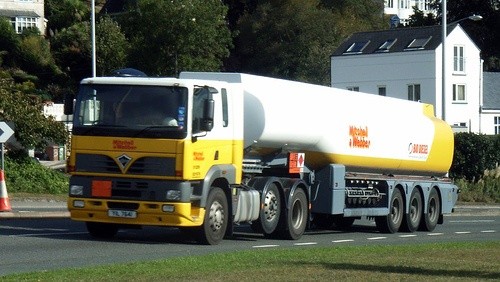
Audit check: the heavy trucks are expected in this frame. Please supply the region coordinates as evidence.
[62,71,460,245]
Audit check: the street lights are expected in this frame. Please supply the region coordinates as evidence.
[441,0,483,128]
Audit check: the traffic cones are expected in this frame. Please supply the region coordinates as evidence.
[0,169,11,212]
